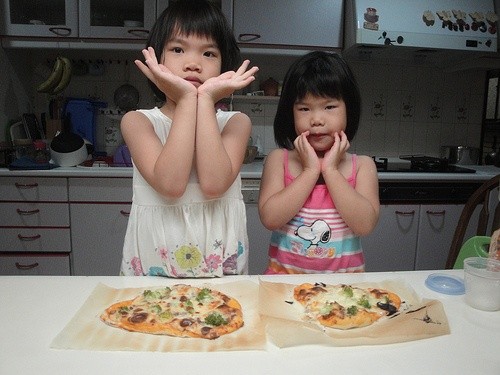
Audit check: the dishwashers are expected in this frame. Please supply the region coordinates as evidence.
[242,178,274,275]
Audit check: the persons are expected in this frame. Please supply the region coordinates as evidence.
[258,50,381,274]
[117,0,259,276]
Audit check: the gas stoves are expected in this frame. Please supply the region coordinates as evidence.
[370,155,476,173]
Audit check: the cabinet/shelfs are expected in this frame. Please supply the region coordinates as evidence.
[359,202,486,271]
[0,174,133,275]
[0,0,346,58]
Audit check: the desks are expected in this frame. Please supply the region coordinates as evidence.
[0,269,499,375]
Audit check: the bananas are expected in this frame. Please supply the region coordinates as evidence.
[36,56,72,94]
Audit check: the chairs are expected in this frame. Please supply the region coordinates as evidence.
[445,174,500,269]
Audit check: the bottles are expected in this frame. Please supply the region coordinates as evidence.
[14,138,34,161]
[250,73,260,94]
[262,77,278,96]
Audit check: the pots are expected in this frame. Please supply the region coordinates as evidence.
[49,133,94,167]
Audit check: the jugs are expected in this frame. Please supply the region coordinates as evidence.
[61,98,108,157]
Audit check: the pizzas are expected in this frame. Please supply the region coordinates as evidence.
[99,284,244,339]
[294,282,401,329]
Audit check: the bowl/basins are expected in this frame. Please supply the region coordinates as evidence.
[440,145,480,165]
[243,146,257,164]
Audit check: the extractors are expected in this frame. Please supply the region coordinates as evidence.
[338,0,498,65]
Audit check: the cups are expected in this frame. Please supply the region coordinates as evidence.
[463,257,500,311]
